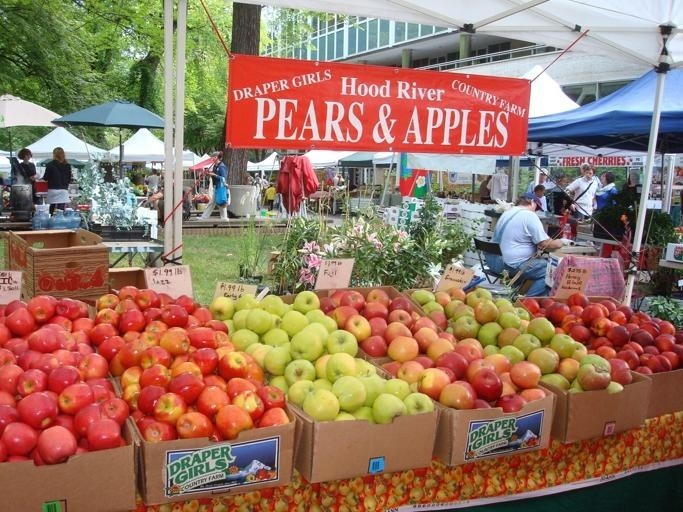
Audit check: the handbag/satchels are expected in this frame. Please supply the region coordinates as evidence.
[214,183,231,206]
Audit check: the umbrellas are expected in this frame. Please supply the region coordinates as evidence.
[0,92,219,179]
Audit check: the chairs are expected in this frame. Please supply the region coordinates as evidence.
[473,238,510,285]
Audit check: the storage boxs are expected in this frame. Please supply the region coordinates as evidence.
[6,227,109,302]
[106,266,147,294]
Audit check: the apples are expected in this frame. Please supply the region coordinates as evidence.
[91,285,289,443]
[319,288,546,414]
[521,293,683,383]
[411,288,624,394]
[0,295,130,466]
[209,291,435,424]
[147,412,683,511]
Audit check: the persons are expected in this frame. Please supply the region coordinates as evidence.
[145,169,160,195]
[44,148,71,216]
[493,193,563,296]
[527,163,642,218]
[480,175,491,203]
[18,149,37,209]
[145,183,163,223]
[247,172,276,210]
[196,152,227,220]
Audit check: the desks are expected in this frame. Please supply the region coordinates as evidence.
[483,208,565,245]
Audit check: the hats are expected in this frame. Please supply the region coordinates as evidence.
[520,193,543,211]
[539,168,548,175]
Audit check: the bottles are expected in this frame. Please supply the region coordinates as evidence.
[563,223,571,240]
